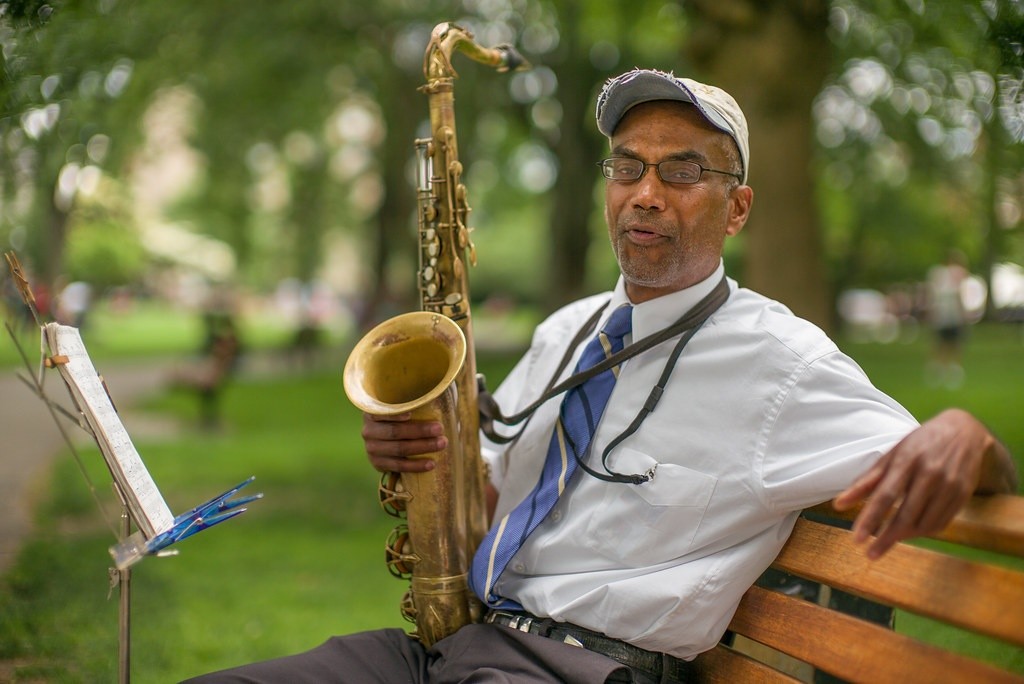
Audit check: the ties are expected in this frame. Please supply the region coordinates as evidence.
[468,306,632,612]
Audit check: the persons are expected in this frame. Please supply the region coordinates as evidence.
[181,69,1018,684]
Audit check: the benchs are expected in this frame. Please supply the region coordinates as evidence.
[686,492,1024,684]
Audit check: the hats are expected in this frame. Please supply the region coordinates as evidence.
[596,69,749,185]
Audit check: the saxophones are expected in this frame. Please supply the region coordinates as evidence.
[340,19,530,652]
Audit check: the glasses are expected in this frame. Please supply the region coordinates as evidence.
[596,157,741,185]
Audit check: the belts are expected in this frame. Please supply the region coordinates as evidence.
[482,613,694,683]
